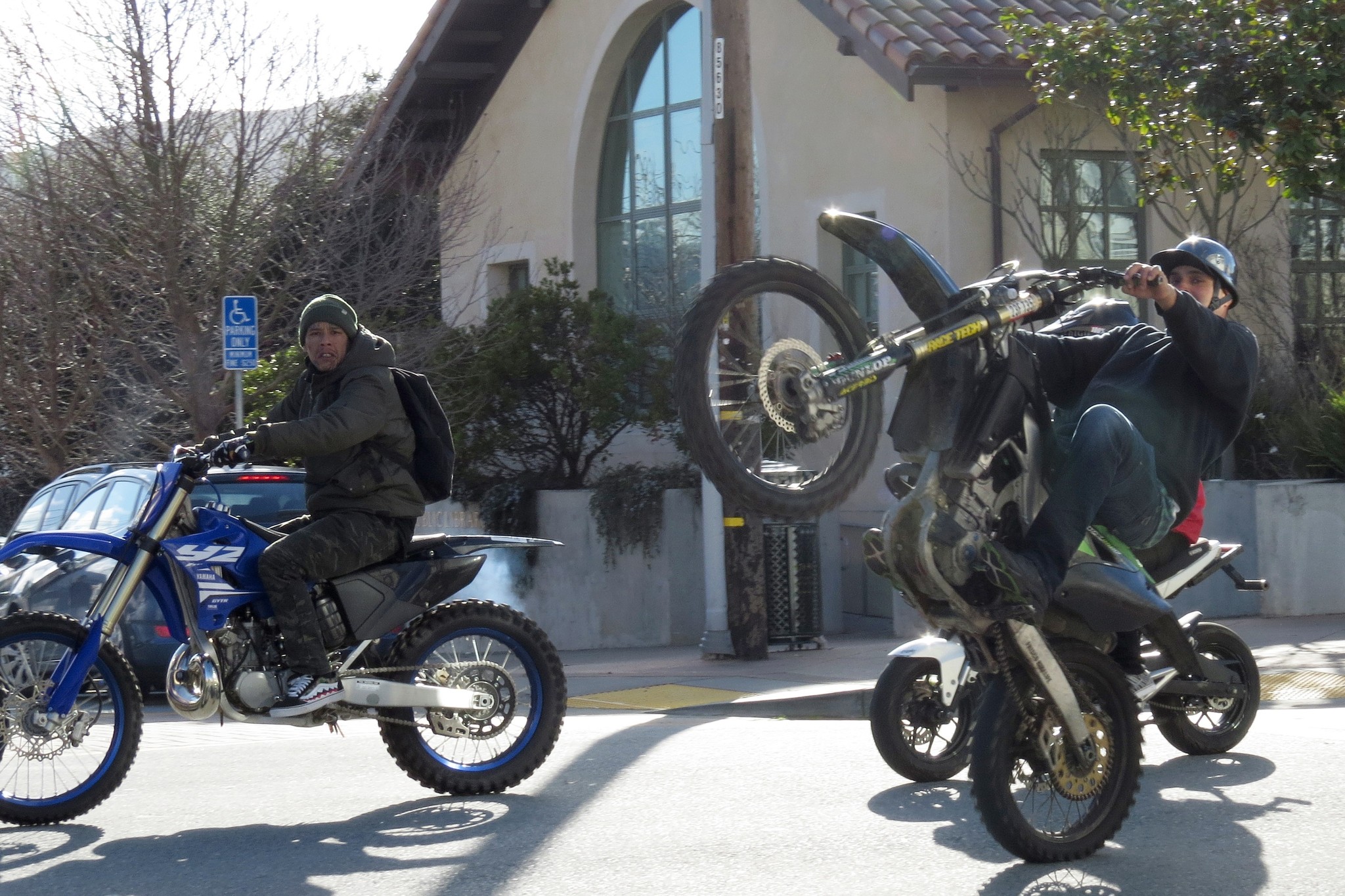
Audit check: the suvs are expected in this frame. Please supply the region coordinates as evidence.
[0,461,308,703]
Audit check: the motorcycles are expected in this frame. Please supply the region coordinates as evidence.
[665,208,1271,867]
[1,443,570,826]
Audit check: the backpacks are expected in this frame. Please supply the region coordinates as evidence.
[323,371,456,502]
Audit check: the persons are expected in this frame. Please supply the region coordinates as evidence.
[173,294,426,718]
[863,236,1260,628]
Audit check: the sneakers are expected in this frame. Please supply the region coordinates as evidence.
[860,527,914,607]
[1094,670,1156,711]
[981,540,1048,628]
[269,673,345,717]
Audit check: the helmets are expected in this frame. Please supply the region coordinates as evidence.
[1152,236,1239,310]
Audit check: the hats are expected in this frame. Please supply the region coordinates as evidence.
[298,293,359,350]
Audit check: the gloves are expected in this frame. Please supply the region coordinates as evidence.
[174,445,201,456]
[210,433,256,468]
